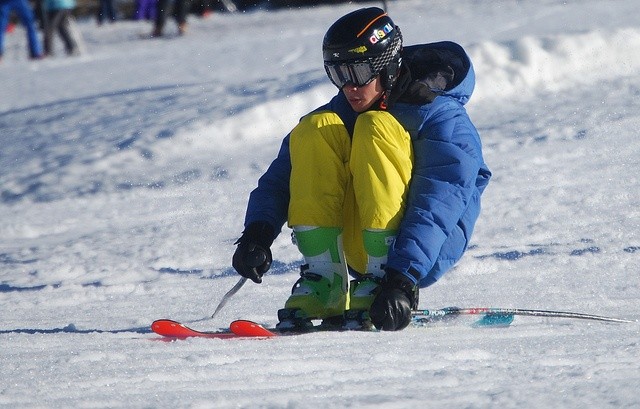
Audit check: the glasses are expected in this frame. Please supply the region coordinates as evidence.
[325,26,403,91]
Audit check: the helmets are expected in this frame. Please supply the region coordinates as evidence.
[323,7,403,91]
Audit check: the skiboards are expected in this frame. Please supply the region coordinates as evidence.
[151,307,514,337]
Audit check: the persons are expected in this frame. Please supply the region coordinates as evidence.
[0,0,45,59]
[42,1,79,58]
[231,8,495,332]
[152,0,190,35]
[98,0,117,25]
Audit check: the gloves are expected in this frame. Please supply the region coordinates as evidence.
[232,224,276,285]
[370,269,418,333]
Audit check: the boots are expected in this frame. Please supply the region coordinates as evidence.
[349,229,399,310]
[285,225,348,320]
